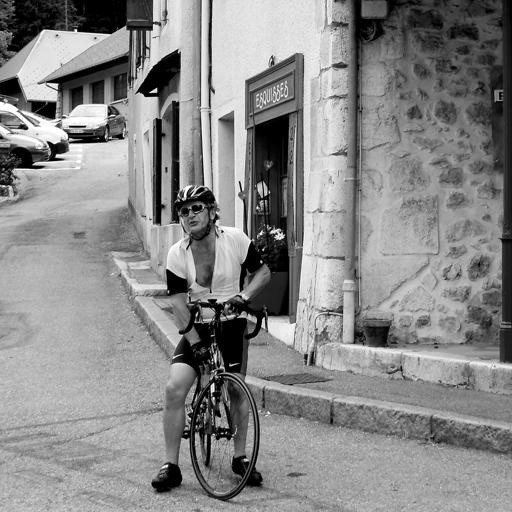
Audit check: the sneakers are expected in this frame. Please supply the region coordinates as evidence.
[151,462,182,492]
[231,455,263,487]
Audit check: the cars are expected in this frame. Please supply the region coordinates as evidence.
[0,101,127,167]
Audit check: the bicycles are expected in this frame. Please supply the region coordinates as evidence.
[179,295,268,503]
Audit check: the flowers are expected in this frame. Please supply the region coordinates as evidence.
[253,225,286,271]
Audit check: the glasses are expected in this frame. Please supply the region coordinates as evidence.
[178,205,208,218]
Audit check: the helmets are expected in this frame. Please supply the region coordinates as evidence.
[173,185,215,211]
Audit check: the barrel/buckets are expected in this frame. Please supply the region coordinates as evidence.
[360,309,394,346]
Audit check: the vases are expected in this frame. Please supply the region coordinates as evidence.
[363,317,390,348]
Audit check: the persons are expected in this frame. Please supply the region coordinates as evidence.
[152,184,272,491]
